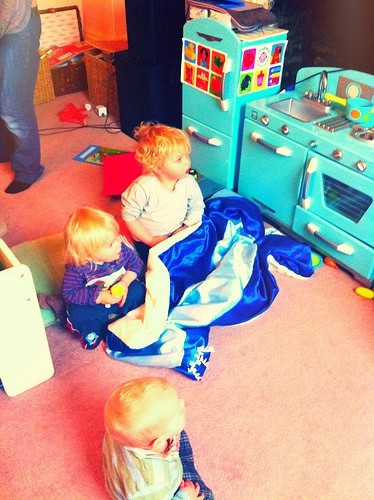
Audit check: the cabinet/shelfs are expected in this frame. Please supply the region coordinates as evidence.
[236,115,374,290]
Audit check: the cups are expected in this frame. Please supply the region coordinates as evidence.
[345,97,374,124]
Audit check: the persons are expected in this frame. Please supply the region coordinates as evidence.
[0,0,45,194]
[121,124,205,263]
[102,377,214,500]
[62,206,146,350]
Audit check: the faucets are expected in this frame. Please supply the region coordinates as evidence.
[315,70,329,105]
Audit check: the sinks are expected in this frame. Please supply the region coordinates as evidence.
[267,97,331,123]
